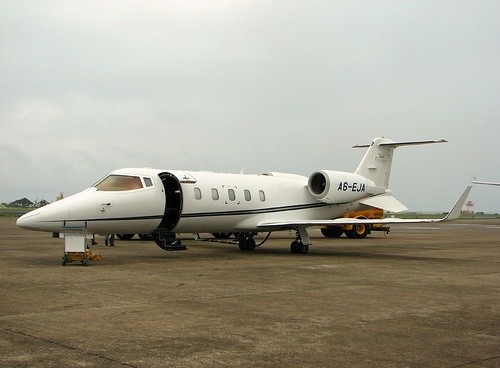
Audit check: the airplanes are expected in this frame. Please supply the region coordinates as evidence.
[17,137,473,266]
